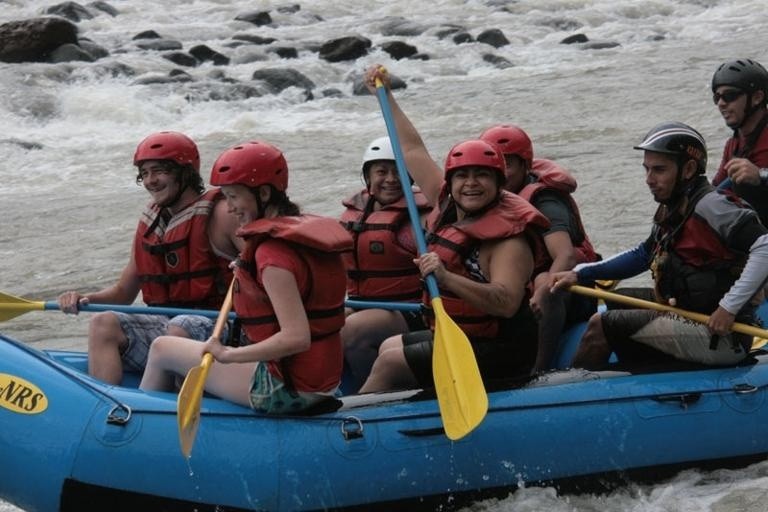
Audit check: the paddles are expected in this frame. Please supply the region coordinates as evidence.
[374,74,488,440]
[0,290,237,323]
[177,274,236,458]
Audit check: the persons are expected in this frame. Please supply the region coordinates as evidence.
[138,141,355,416]
[710,58,768,234]
[479,124,597,379]
[549,121,768,370]
[57,131,246,393]
[339,136,435,393]
[358,65,551,394]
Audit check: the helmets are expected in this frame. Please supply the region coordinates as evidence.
[480,125,533,161]
[361,136,414,186]
[712,59,768,105]
[134,132,200,177]
[211,141,288,191]
[634,121,707,174]
[444,140,507,180]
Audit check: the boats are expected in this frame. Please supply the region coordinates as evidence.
[0,303,768,511]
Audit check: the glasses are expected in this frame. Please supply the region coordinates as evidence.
[713,90,744,104]
